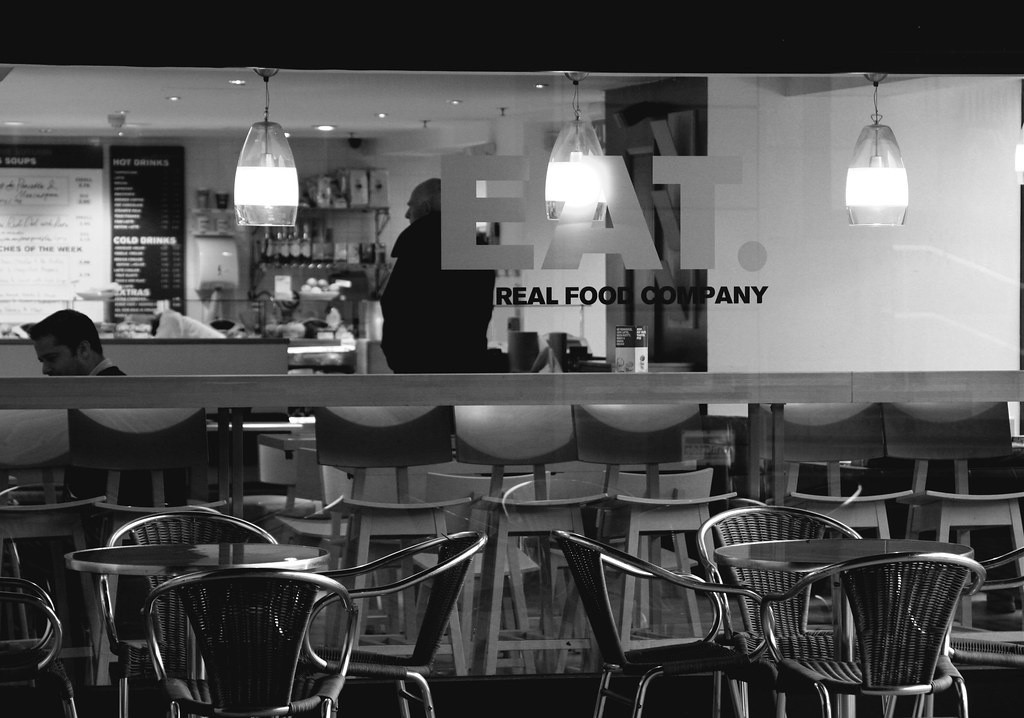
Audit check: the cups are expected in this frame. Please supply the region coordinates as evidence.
[274,276,294,300]
[197,187,231,235]
[335,241,386,265]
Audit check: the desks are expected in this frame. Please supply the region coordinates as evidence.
[1,376,1024,511]
[63,541,330,718]
[706,538,980,718]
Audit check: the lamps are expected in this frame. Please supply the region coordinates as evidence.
[542,73,609,228]
[230,69,301,231]
[841,74,917,231]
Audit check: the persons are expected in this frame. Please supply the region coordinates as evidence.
[28,309,186,645]
[381,178,495,373]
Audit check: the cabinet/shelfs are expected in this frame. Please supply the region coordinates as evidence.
[249,168,390,303]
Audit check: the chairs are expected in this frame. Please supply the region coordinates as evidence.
[0,405,1024,717]
[0,575,79,718]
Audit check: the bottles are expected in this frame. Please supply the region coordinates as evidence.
[305,168,349,208]
[259,222,333,262]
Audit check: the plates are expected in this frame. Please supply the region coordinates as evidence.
[299,291,341,301]
